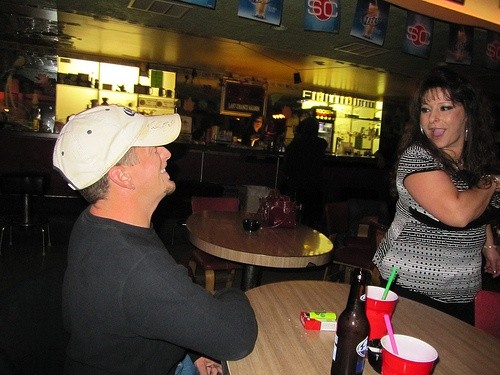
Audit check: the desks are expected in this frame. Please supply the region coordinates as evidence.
[222,278,500,375]
[185,211,335,295]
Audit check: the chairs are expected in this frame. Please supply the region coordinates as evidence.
[475,290,500,338]
[187,195,244,293]
[323,201,374,284]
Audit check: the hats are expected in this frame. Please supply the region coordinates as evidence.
[52,105,181,191]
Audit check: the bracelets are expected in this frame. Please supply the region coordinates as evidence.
[483,245,497,249]
[479,174,499,184]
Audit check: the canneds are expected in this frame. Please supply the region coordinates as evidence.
[33,119,39,132]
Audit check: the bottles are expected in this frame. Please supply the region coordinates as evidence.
[44,105,55,133]
[32,108,43,132]
[330,268,371,375]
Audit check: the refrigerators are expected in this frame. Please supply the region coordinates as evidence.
[309,105,336,154]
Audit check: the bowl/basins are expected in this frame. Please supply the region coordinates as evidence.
[243,219,260,232]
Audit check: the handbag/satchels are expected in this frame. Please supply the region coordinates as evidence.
[256,190,296,229]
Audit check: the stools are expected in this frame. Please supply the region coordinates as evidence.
[0,173,53,257]
[157,197,190,249]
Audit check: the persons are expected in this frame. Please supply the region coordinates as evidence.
[280,116,328,234]
[371,65,500,327]
[241,112,265,147]
[51,105,258,375]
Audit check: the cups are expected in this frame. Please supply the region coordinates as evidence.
[365,285,399,341]
[380,333,439,375]
